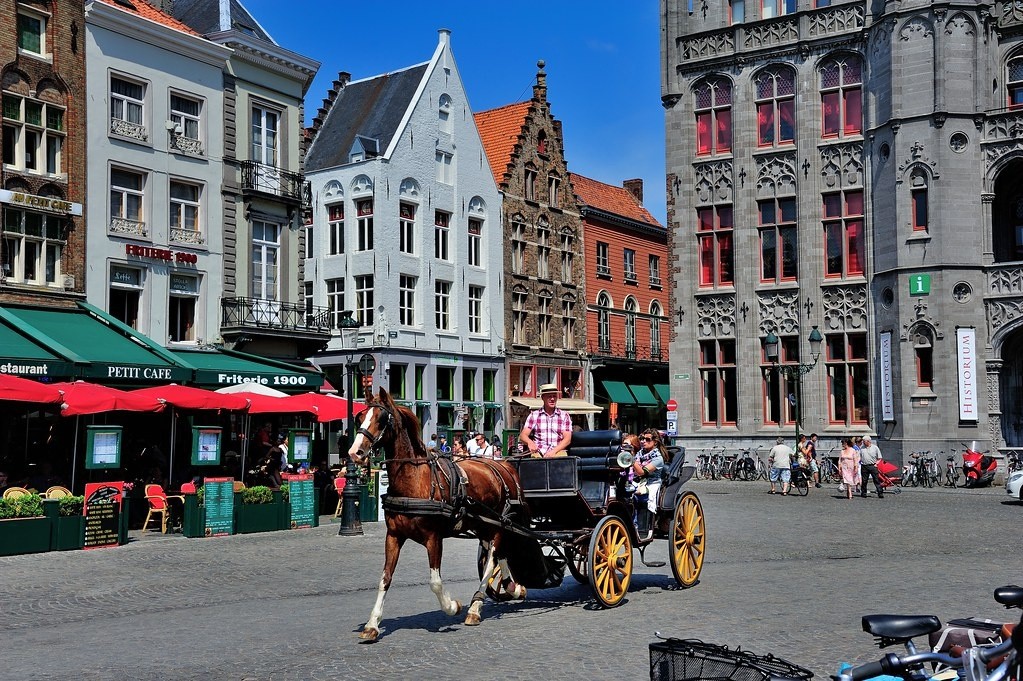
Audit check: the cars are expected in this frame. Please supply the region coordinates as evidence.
[1005,470,1023,499]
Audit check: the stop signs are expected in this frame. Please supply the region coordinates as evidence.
[667,400,677,410]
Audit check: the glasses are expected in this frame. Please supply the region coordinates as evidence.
[547,395,557,398]
[641,437,653,442]
[476,439,480,441]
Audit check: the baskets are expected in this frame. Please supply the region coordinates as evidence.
[649,642,813,681]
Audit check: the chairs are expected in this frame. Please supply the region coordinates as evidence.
[234,480,245,492]
[181,482,196,492]
[142,483,185,536]
[46,486,73,499]
[332,478,347,516]
[3,487,32,500]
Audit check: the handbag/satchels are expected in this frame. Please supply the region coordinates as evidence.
[837,475,845,492]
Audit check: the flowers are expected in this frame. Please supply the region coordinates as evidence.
[122,481,134,491]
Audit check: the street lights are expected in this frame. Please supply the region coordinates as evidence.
[759,326,823,458]
[337,310,364,536]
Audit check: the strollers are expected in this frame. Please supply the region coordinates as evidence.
[875,460,902,495]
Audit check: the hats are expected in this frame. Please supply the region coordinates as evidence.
[777,437,784,443]
[438,434,445,438]
[855,437,863,443]
[539,384,562,396]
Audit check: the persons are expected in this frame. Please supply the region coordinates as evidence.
[337,427,350,463]
[426,433,523,458]
[617,427,669,530]
[837,435,885,500]
[521,383,572,457]
[254,421,292,486]
[767,437,795,496]
[797,433,822,489]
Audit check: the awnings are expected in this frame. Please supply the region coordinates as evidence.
[627,384,659,408]
[150,344,324,481]
[594,377,636,407]
[513,397,602,415]
[0,300,197,495]
[652,383,670,406]
[304,365,338,394]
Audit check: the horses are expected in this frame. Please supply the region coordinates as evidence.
[347,385,528,641]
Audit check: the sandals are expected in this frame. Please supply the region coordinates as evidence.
[780,492,787,496]
[768,489,775,494]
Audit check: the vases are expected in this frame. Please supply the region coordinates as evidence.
[122,491,126,497]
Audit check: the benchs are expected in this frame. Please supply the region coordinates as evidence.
[629,458,676,515]
[563,428,627,478]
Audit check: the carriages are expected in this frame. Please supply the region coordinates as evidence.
[347,387,706,643]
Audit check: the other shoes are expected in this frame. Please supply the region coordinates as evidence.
[878,493,884,498]
[815,483,822,488]
[861,493,867,498]
[856,489,860,492]
[848,494,852,500]
[791,484,795,488]
[808,485,812,488]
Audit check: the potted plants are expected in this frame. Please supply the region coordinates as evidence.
[0,492,85,556]
[197,483,291,536]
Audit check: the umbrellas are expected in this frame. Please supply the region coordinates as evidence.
[284,392,369,454]
[227,392,321,481]
[0,373,167,492]
[128,381,251,486]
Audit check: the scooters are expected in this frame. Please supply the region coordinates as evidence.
[960,442,998,488]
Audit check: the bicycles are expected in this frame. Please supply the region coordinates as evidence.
[692,445,959,489]
[1006,451,1023,474]
[779,463,812,495]
[649,585,1023,681]
